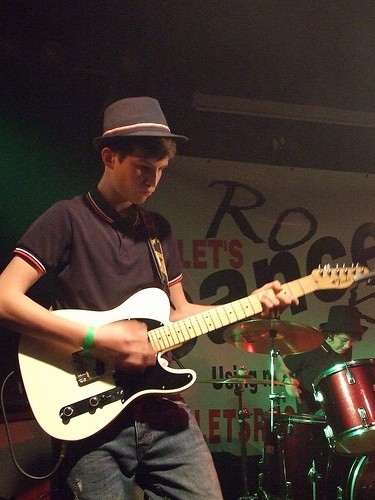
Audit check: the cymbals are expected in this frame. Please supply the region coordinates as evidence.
[195,378,290,387]
[226,319,324,354]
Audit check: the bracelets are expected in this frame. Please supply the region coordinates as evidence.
[280,374,294,387]
[82,326,96,356]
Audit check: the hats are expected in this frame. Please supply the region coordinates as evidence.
[319,305,368,333]
[93,97,189,145]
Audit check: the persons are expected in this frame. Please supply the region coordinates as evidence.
[276,305,368,500]
[0,97,299,500]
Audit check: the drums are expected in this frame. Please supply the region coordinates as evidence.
[262,410,327,500]
[326,454,375,500]
[315,357,375,455]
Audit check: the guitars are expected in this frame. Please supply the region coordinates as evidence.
[18,263,369,442]
